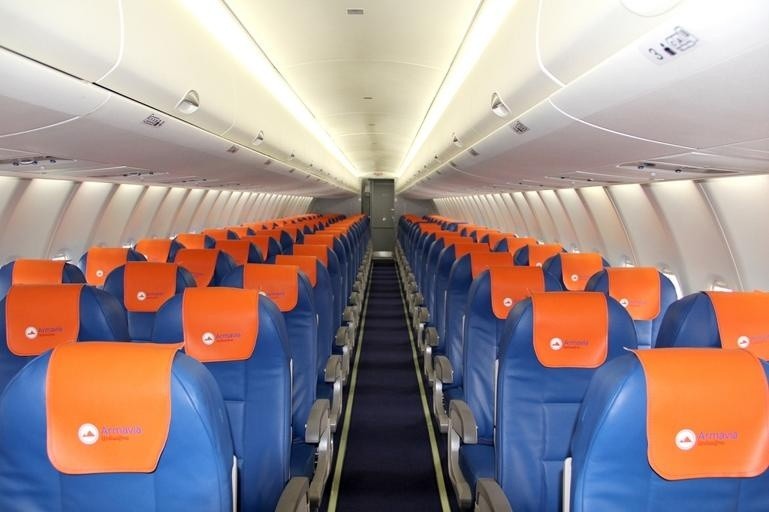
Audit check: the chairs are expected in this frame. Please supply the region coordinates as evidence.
[393,208,767,511]
[0,211,374,512]
